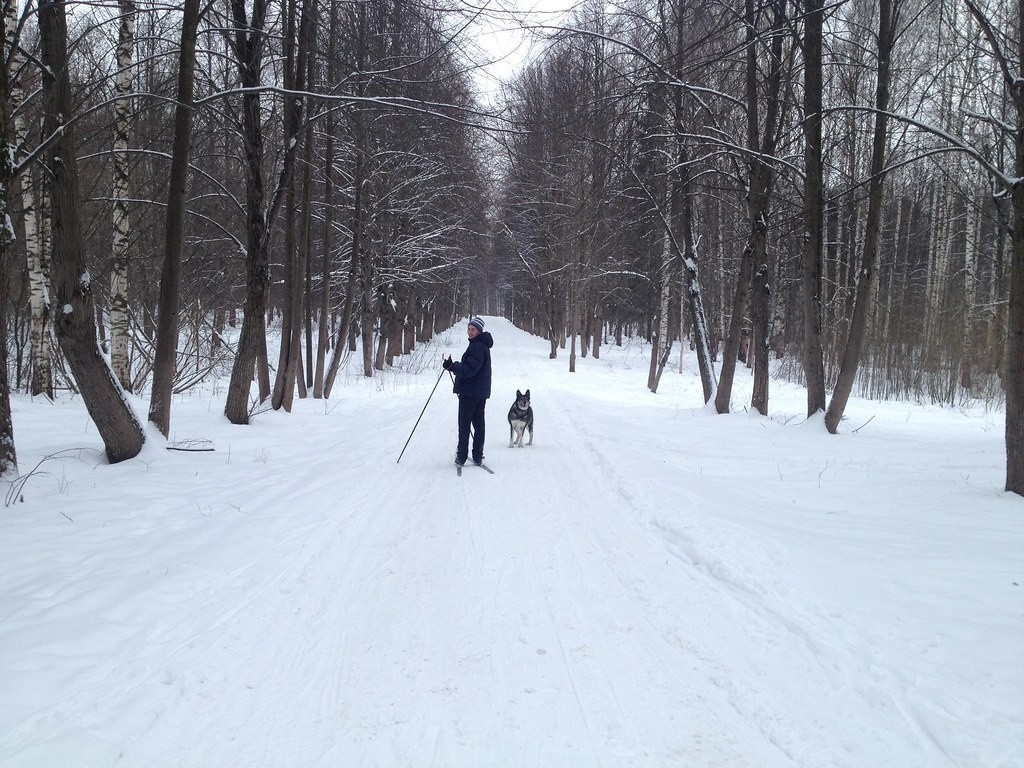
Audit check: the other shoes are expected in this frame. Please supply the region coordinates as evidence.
[473,459,482,466]
[455,458,464,465]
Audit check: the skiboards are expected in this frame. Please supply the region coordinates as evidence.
[457,456,495,477]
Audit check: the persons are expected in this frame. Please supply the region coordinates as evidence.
[443,318,493,466]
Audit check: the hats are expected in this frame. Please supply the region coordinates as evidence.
[468,317,485,331]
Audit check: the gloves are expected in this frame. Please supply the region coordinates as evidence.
[443,358,456,372]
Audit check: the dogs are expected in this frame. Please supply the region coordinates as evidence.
[507,389,534,449]
